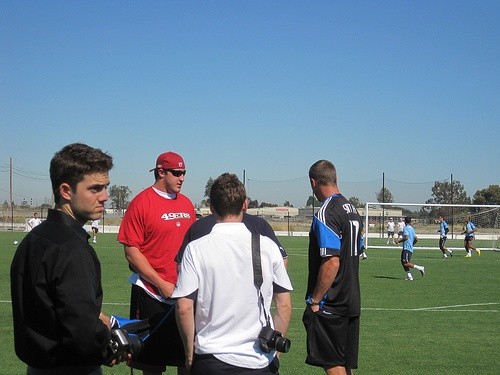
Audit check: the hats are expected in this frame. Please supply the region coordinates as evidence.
[149,152,185,172]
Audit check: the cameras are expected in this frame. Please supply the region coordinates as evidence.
[103,329,145,365]
[258,326,291,353]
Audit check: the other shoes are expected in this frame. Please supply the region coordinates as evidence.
[363,252,367,258]
[393,242,395,244]
[93,236,96,239]
[93,241,96,244]
[359,253,363,259]
[387,241,389,244]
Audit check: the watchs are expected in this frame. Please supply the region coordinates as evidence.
[309,298,320,305]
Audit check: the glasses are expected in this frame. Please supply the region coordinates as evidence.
[164,169,186,177]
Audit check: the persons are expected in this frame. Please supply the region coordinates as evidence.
[91,220,100,243]
[386,216,425,281]
[436,215,453,258]
[302,160,362,375]
[28,213,42,229]
[360,228,368,259]
[11,143,132,375]
[117,152,197,375]
[171,173,294,375]
[460,216,480,257]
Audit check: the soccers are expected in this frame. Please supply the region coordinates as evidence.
[13,240,19,245]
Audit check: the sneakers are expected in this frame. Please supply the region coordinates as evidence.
[419,265,424,277]
[476,249,480,256]
[464,253,471,257]
[450,251,453,257]
[403,276,413,280]
[441,256,448,259]
